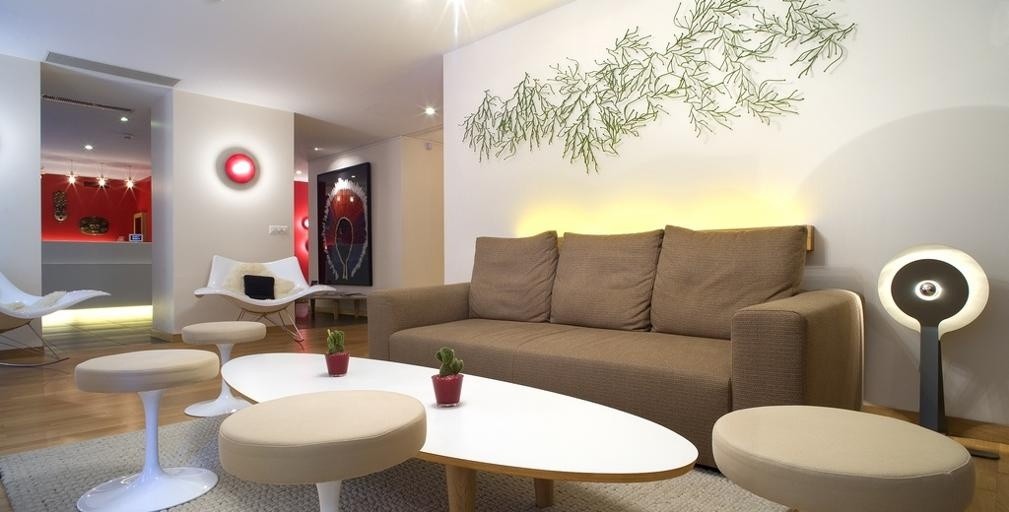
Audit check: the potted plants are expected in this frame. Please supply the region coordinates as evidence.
[323,328,351,377]
[430,346,465,411]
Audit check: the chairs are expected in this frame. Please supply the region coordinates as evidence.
[0,271,112,369]
[194,254,337,344]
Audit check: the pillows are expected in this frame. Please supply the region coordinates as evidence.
[242,273,275,300]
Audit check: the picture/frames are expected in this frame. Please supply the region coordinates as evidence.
[316,161,374,287]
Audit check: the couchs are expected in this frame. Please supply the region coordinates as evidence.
[365,225,868,470]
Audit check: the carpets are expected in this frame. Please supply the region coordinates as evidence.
[1,413,794,512]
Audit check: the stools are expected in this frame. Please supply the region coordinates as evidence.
[711,404,976,512]
[181,320,267,418]
